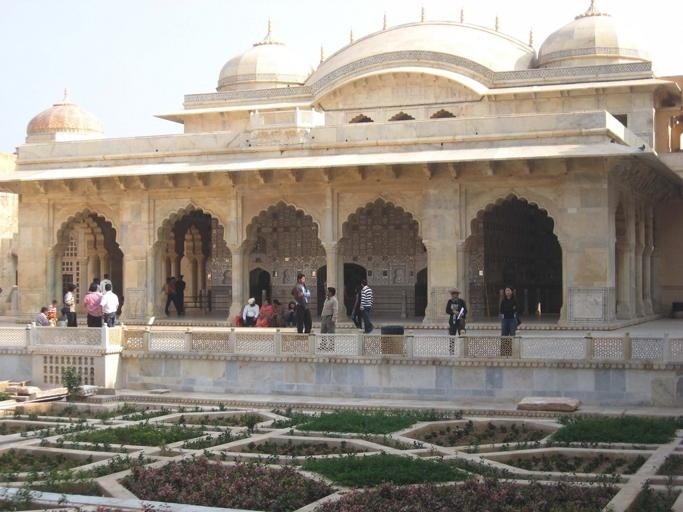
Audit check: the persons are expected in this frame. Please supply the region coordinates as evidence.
[499,285,518,356]
[99,283,119,326]
[359,279,374,333]
[92,278,101,293]
[35,307,50,326]
[444,286,467,356]
[283,300,297,327]
[82,282,103,326]
[63,284,77,326]
[159,274,186,317]
[291,273,312,334]
[99,274,112,293]
[241,297,259,327]
[271,299,283,327]
[46,299,57,326]
[316,286,338,352]
[254,298,272,327]
[350,286,362,329]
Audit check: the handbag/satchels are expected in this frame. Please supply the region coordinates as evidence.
[60,307,70,316]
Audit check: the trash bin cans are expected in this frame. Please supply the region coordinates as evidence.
[673,302,683,318]
[381,326,404,354]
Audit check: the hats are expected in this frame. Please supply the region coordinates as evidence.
[448,287,461,294]
[247,297,256,305]
